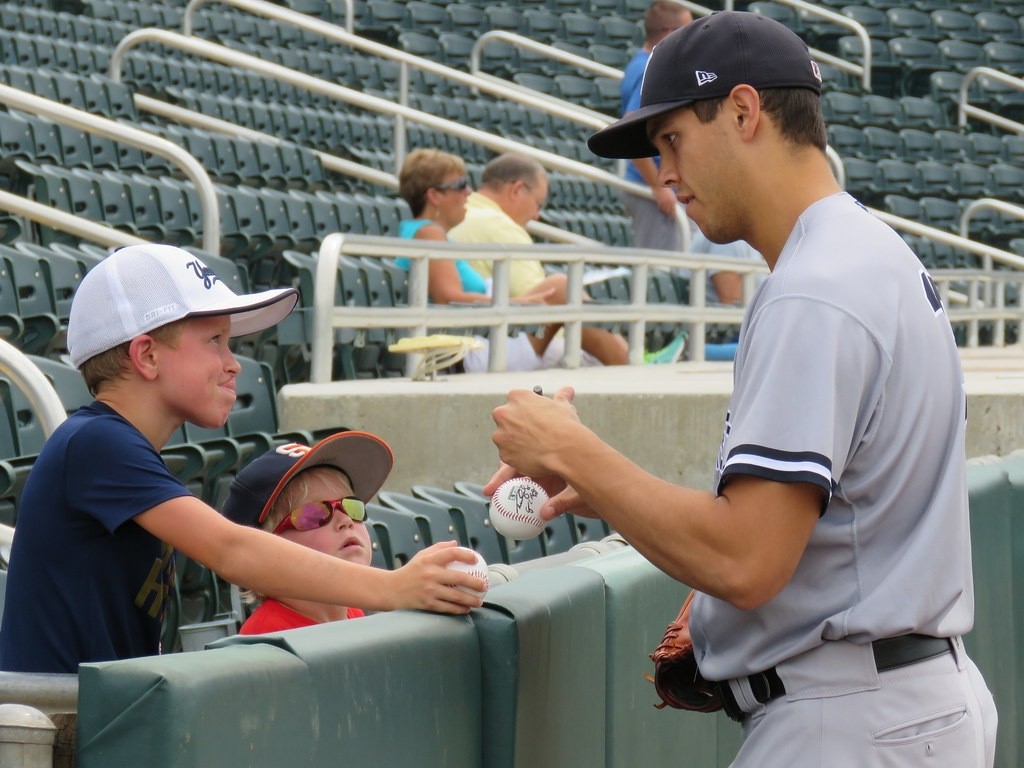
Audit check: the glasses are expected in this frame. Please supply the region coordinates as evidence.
[434,175,469,193]
[523,182,544,212]
[274,496,367,537]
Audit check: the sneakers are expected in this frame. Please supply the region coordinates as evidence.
[642,328,688,363]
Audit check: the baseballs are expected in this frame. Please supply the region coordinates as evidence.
[488,478,550,541]
[443,546,489,600]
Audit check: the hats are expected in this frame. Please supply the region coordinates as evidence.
[218,432,393,525]
[67,241,300,368]
[587,9,822,158]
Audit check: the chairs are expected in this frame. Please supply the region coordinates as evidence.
[231,353,351,443]
[412,483,502,565]
[376,491,461,546]
[539,511,573,556]
[453,480,543,565]
[0,401,41,516]
[365,502,426,570]
[0,0,1024,391]
[187,419,314,448]
[565,513,605,545]
[0,370,55,458]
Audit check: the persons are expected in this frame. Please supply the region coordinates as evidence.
[221,431,395,636]
[447,153,608,366]
[679,226,767,305]
[1,243,487,675]
[616,0,695,251]
[490,13,1002,768]
[395,149,688,377]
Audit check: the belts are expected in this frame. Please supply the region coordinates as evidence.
[715,628,955,721]
